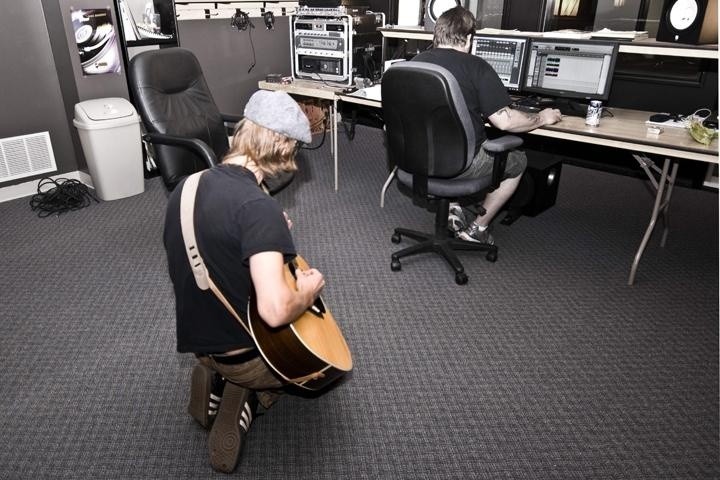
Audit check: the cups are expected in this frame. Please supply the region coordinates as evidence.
[152,14,161,34]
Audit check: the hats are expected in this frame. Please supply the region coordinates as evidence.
[243,90,312,144]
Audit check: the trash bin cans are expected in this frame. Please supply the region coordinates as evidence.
[72,97,145,201]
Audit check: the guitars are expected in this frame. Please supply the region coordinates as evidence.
[247,179,354,392]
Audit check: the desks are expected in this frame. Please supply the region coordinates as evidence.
[340,82,719,287]
[258,73,374,192]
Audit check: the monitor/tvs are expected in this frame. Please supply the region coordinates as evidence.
[520,39,619,117]
[470,33,530,103]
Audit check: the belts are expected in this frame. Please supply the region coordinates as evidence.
[196,348,260,364]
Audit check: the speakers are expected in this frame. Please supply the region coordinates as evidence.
[424,0,504,36]
[655,0,718,45]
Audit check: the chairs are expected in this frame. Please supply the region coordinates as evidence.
[378,60,525,285]
[127,47,299,198]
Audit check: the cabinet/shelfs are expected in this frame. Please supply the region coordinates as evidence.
[114,0,180,179]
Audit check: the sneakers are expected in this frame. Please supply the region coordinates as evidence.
[448,207,495,245]
[209,382,257,473]
[189,365,223,429]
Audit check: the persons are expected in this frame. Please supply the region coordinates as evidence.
[410,7,561,247]
[163,88,327,475]
[483,3,485,5]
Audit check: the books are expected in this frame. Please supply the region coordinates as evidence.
[589,28,649,42]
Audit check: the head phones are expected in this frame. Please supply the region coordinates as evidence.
[230,10,255,31]
